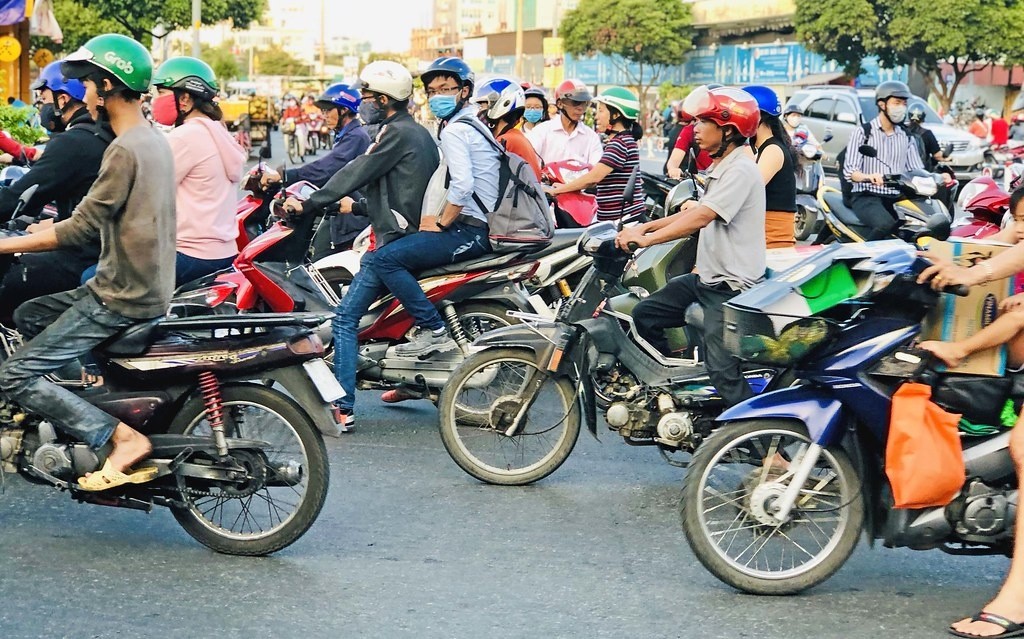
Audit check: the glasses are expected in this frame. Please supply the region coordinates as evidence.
[561,98,589,107]
[320,106,340,115]
[523,104,544,111]
[425,86,460,99]
[359,95,379,102]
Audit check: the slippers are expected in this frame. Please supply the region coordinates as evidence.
[947,607,1023,637]
[78,455,161,495]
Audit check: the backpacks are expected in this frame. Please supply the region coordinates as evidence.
[439,116,556,255]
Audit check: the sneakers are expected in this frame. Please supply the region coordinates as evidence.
[380,388,425,405]
[329,407,355,432]
[393,328,461,358]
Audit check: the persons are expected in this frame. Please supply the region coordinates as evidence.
[0,32,1024,639]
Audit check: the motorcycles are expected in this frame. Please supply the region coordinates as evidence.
[679,243,1024,594]
[0,183,349,555]
[947,174,1024,256]
[0,136,835,409]
[207,161,552,427]
[439,165,843,495]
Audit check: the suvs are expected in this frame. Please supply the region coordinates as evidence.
[784,85,984,190]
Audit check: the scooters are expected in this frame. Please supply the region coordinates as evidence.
[966,139,1024,178]
[789,133,833,240]
[302,112,326,155]
[321,117,337,150]
[931,143,955,225]
[809,143,956,277]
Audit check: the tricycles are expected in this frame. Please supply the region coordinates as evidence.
[218,96,272,158]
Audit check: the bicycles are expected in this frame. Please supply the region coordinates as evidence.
[281,115,306,163]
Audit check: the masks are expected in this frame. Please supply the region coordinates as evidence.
[356,99,391,129]
[425,88,464,119]
[147,95,186,128]
[519,107,545,124]
[38,103,66,138]
[787,116,801,128]
[886,104,906,124]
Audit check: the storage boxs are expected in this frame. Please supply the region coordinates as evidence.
[760,263,856,335]
[924,239,1018,377]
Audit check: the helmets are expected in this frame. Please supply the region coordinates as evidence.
[670,86,761,139]
[590,86,640,122]
[57,31,154,98]
[474,78,527,129]
[148,55,220,99]
[311,85,359,113]
[345,60,414,103]
[419,55,475,99]
[29,63,89,107]
[525,88,546,105]
[873,78,932,129]
[738,83,780,119]
[552,79,591,110]
[782,103,802,120]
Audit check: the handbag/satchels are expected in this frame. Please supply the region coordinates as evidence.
[886,382,963,514]
